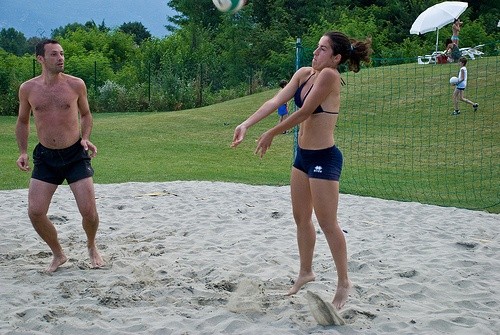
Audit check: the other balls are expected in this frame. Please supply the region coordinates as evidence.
[449,76,458,85]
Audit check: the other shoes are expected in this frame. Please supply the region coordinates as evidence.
[451,110,460,115]
[473,104,479,111]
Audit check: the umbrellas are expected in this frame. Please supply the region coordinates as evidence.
[409,1,469,55]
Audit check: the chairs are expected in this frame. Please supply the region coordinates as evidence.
[418,44,485,65]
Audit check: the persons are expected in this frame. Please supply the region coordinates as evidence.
[15,40,105,272]
[444,39,461,61]
[231,31,372,310]
[451,18,463,46]
[277,80,290,134]
[450,57,479,115]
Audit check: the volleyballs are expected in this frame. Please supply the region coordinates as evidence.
[211,0,246,14]
